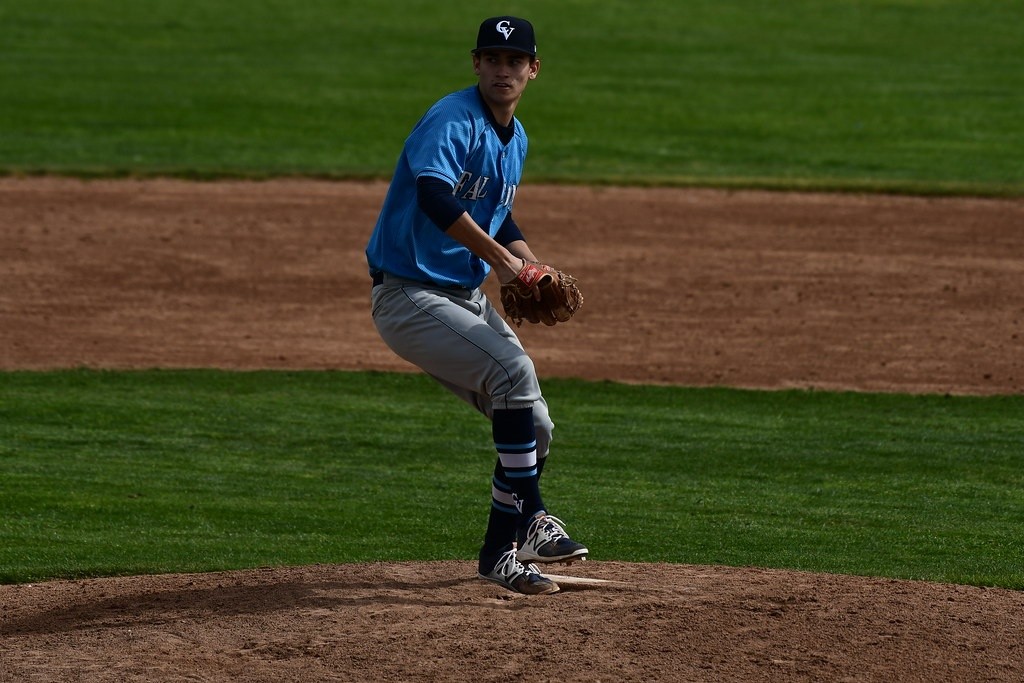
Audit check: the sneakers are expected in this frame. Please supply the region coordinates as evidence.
[516,510,589,562]
[477,542,560,594]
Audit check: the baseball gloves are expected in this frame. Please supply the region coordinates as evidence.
[500,261,582,326]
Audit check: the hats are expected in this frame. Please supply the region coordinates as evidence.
[470,16,537,55]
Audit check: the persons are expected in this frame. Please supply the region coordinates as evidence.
[365,14,588,595]
